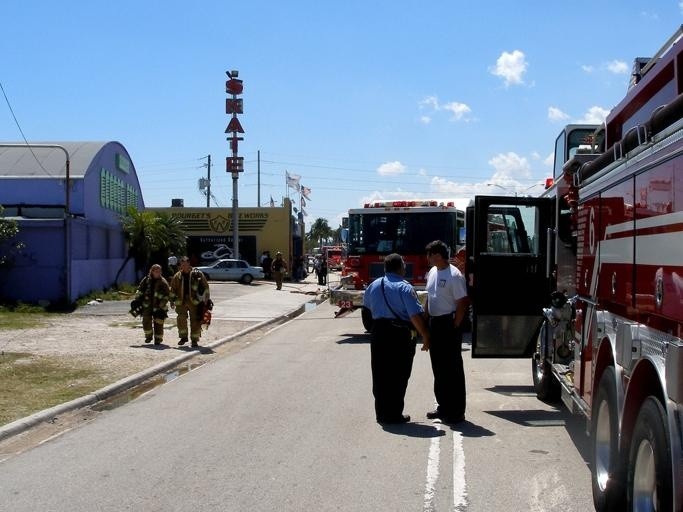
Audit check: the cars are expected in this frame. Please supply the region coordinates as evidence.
[192,258,265,284]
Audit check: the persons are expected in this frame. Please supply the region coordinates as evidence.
[258,249,328,290]
[422,239,468,424]
[167,252,178,274]
[167,256,211,348]
[360,253,430,426]
[134,264,170,345]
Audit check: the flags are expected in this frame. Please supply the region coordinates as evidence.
[286,172,312,217]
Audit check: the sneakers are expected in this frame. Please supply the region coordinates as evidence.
[145,337,152,343]
[191,341,198,348]
[376,415,410,426]
[178,338,188,345]
[426,410,465,424]
[155,339,163,345]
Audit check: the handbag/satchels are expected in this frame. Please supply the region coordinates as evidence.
[197,304,211,331]
[128,300,144,318]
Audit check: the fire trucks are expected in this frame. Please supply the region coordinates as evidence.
[467,24,683,511]
[322,244,343,271]
[331,199,528,333]
